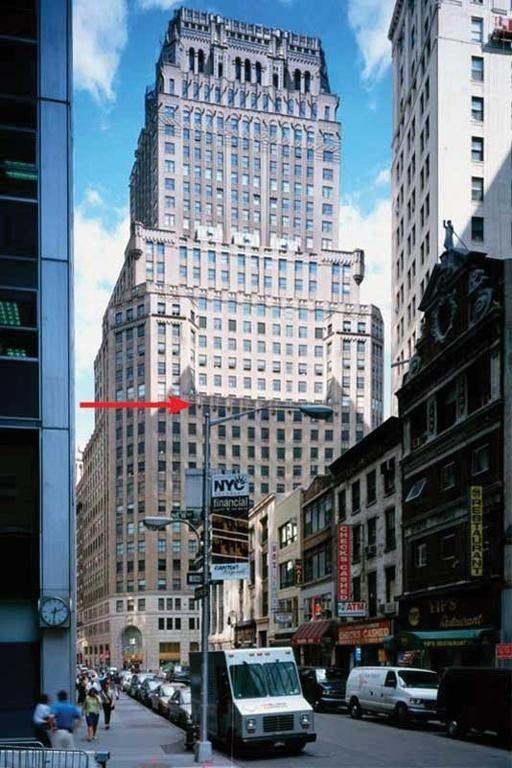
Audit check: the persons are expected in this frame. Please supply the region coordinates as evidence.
[443,219,453,250]
[32,659,125,751]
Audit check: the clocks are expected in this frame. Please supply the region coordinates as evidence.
[37,593,70,629]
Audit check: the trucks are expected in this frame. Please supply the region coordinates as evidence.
[188,647,317,754]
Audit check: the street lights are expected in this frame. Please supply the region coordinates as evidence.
[139,403,334,743]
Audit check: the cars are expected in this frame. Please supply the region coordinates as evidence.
[107,663,193,730]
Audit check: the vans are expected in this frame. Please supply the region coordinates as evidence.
[296,664,348,713]
[436,666,511,748]
[345,666,441,726]
